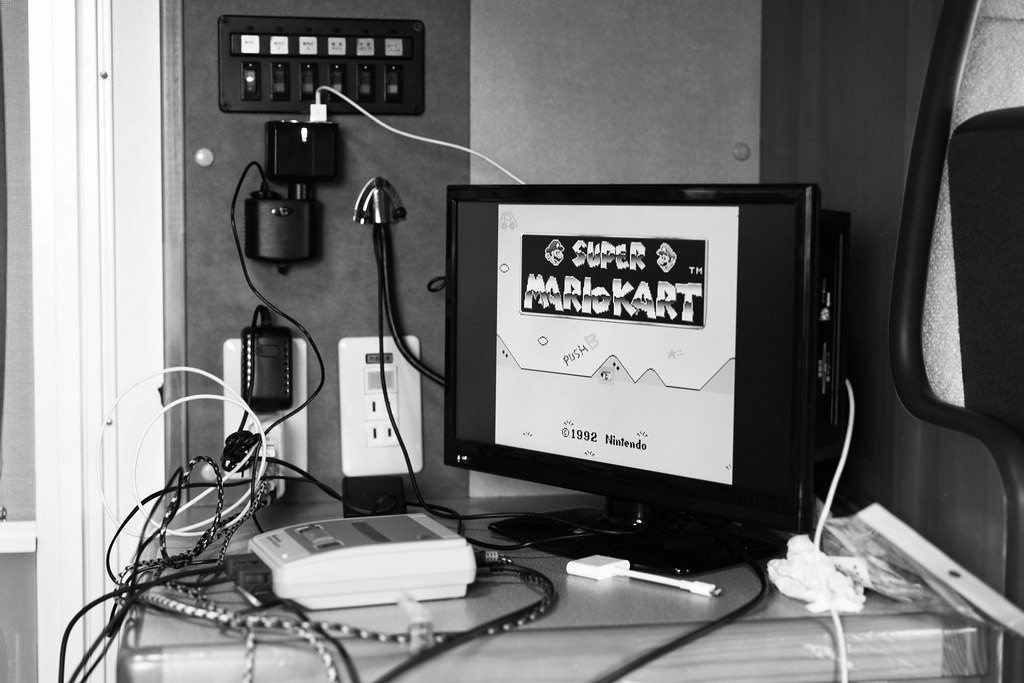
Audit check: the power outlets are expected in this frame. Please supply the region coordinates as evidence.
[339,337,424,478]
[224,340,308,479]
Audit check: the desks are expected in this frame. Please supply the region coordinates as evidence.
[110,495,993,683]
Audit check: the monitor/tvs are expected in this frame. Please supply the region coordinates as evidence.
[444,183,850,575]
